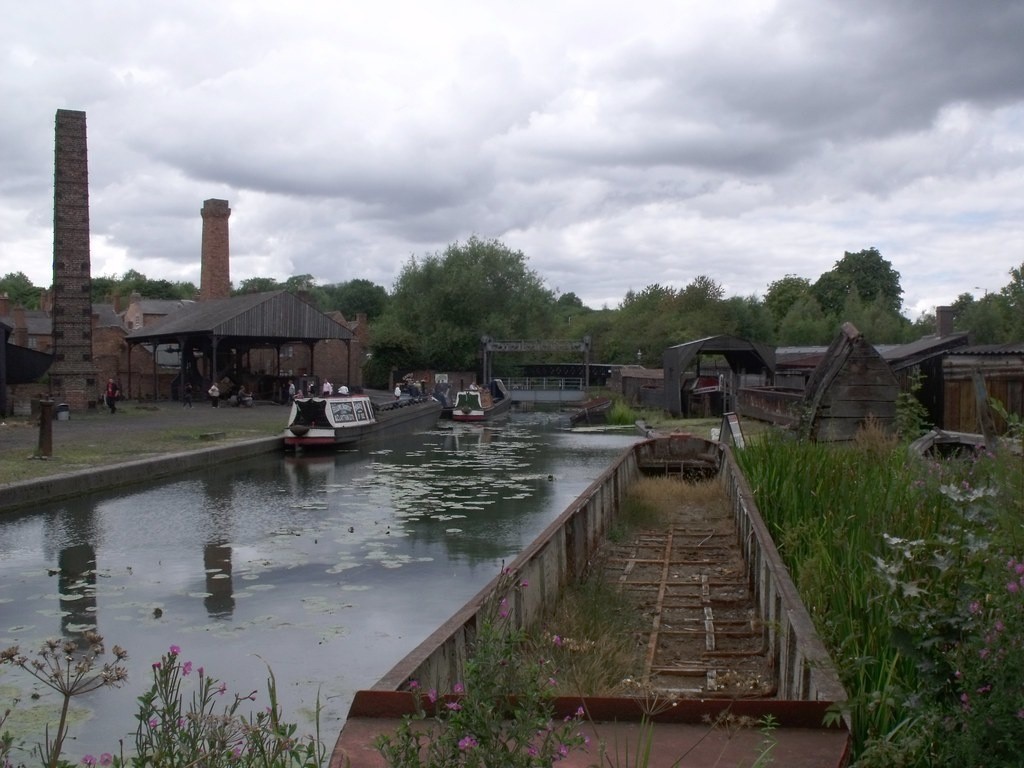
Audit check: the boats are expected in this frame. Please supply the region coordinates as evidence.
[328,433,851,768]
[284,393,444,444]
[452,378,512,421]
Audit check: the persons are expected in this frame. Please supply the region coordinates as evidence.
[287,380,315,406]
[238,386,252,406]
[183,383,192,408]
[208,383,220,408]
[106,378,119,410]
[394,384,401,400]
[322,378,331,396]
[408,380,445,397]
[338,384,348,394]
